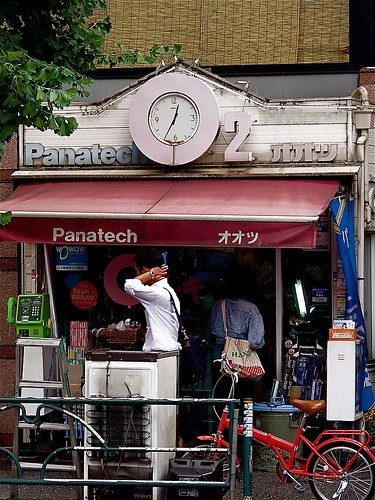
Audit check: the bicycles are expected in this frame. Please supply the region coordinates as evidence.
[177,351,375,500]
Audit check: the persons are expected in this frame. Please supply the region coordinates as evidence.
[124,247,183,352]
[210,275,266,460]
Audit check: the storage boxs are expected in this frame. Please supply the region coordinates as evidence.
[310,286,329,303]
[329,328,357,341]
[324,340,365,423]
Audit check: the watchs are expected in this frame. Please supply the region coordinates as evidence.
[147,271,156,280]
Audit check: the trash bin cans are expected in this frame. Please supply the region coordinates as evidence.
[253,398,304,471]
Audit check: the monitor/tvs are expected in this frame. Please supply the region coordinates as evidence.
[292,279,308,319]
[167,458,223,500]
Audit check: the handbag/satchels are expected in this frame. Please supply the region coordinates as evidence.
[178,328,200,362]
[220,336,265,381]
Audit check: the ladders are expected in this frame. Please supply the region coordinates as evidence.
[9,337,84,499]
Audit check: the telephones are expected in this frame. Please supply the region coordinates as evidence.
[6,294,50,339]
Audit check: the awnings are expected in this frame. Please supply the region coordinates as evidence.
[0,178,342,249]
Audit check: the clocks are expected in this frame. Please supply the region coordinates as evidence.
[146,94,201,146]
